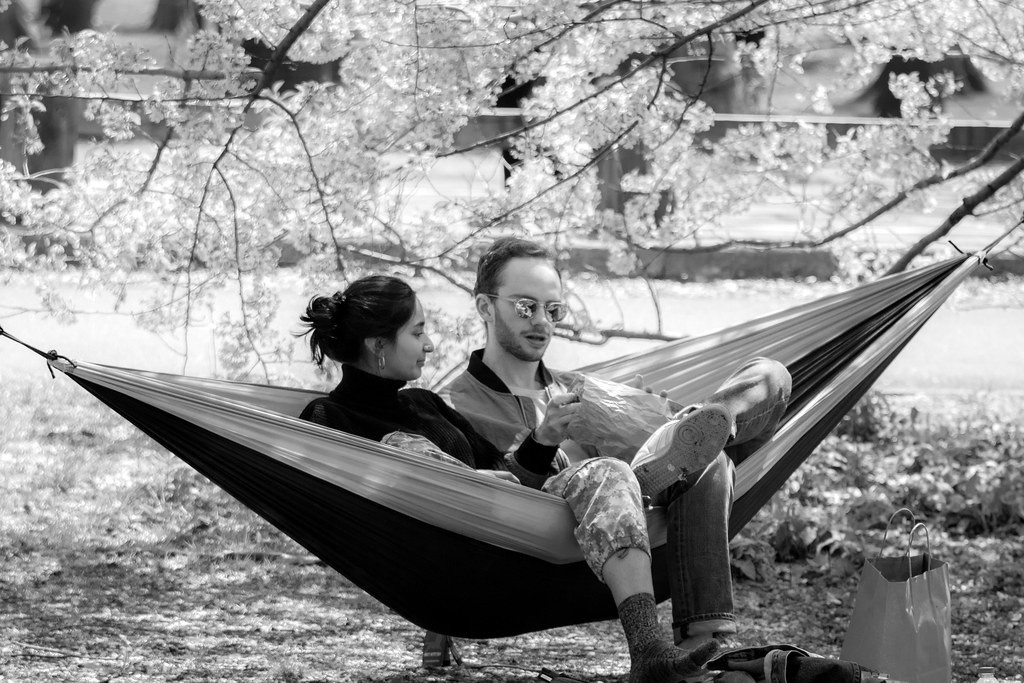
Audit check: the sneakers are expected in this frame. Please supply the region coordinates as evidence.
[630,404,732,500]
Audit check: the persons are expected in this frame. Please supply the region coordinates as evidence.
[437,238,793,652]
[295,275,718,683]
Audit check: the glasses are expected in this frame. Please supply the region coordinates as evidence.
[483,293,570,325]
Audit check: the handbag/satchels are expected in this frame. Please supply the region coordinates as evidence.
[840,509,954,683]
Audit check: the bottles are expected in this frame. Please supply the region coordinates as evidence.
[975,666,999,683]
